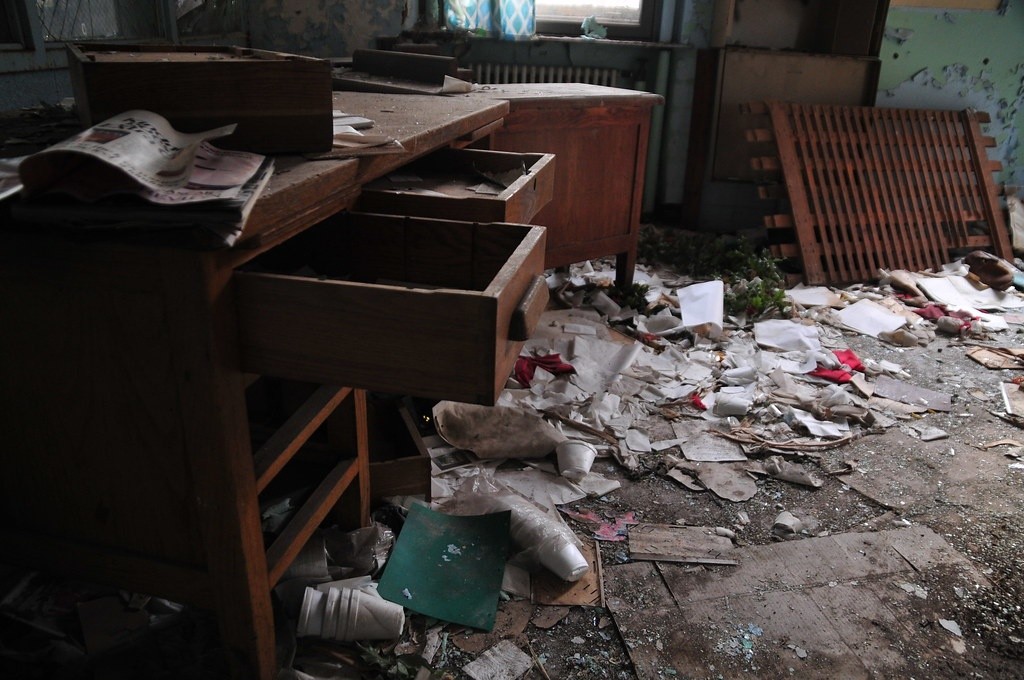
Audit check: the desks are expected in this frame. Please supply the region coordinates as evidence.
[333,67,665,294]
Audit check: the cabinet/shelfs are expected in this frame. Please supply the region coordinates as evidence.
[0,93,554,680]
[682,42,882,235]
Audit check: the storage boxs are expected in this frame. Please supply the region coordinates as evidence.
[66,42,333,155]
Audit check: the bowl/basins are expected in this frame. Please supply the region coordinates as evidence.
[556,440,598,479]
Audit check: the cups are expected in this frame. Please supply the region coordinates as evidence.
[296,587,405,640]
[489,494,589,583]
